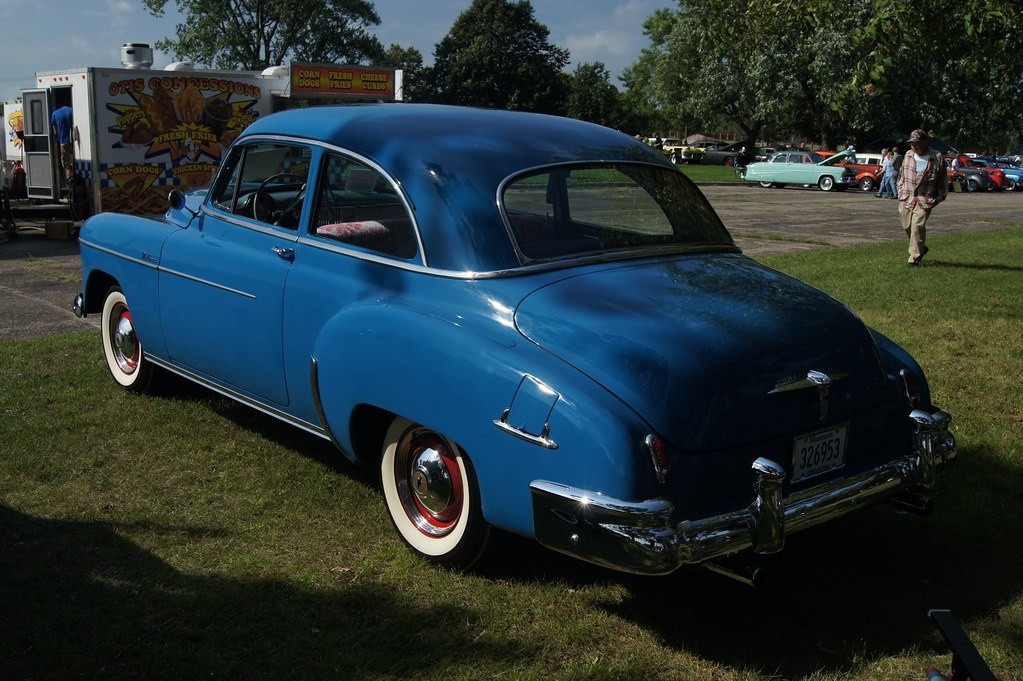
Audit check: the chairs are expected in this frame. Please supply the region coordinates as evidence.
[315,220,390,256]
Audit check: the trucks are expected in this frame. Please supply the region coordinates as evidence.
[0,43,408,217]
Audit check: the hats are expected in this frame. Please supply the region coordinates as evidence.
[906,130,925,144]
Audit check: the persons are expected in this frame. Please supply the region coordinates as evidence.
[884,152,894,197]
[897,129,949,266]
[874,148,891,197]
[11,160,24,176]
[952,153,960,171]
[891,146,903,180]
[652,132,663,152]
[51,95,72,182]
[736,145,746,178]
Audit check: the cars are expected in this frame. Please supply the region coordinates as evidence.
[69,102,954,590]
[631,131,1023,196]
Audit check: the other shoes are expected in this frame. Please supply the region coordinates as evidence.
[873,194,898,200]
[58,196,73,203]
[908,247,929,267]
